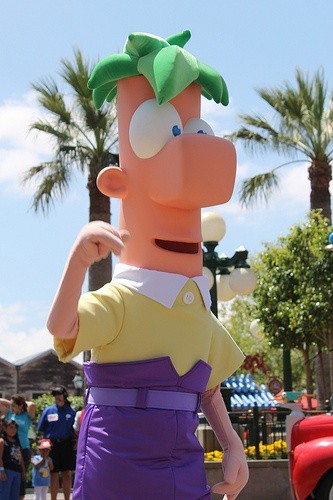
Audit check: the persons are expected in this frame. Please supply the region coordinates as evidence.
[37,385,84,500]
[0,416,27,500]
[31,438,54,500]
[0,395,36,500]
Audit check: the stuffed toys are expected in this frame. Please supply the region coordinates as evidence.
[46,27,251,500]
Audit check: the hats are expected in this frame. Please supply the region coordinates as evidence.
[37,439,53,449]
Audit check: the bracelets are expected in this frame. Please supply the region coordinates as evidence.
[0,466,5,472]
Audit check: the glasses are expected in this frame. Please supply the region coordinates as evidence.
[51,392,63,396]
[50,386,67,395]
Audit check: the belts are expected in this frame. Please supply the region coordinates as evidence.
[84,388,199,413]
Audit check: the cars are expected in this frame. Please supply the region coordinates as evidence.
[289,410,333,500]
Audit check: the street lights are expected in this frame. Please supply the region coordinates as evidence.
[200,211,251,421]
